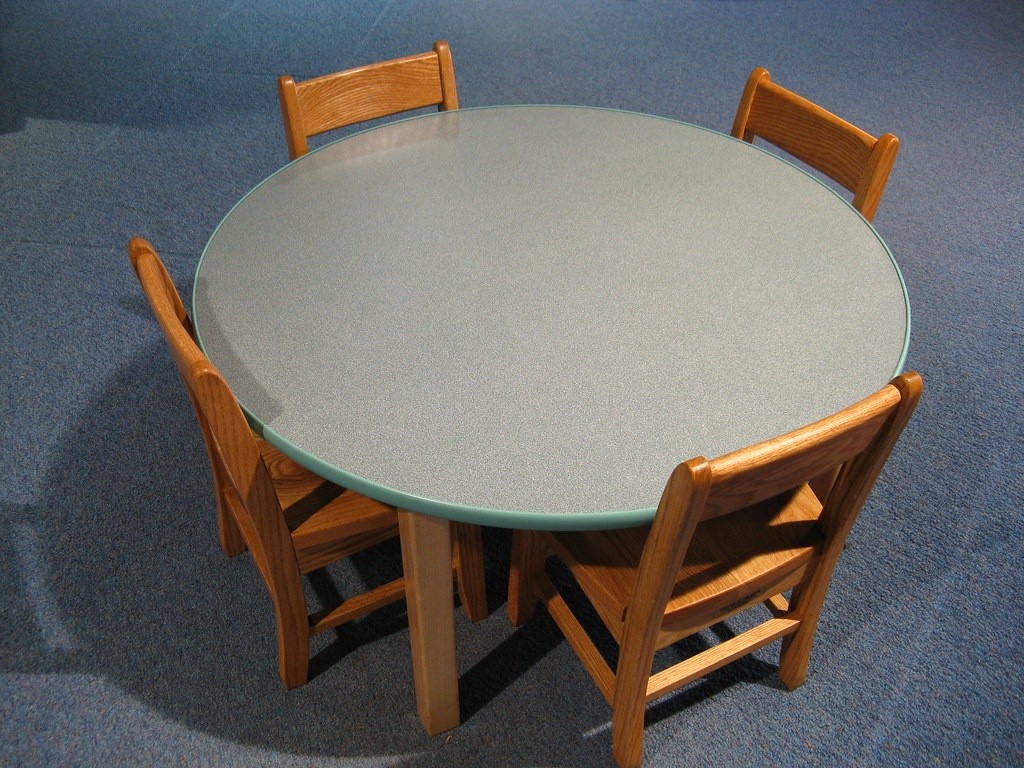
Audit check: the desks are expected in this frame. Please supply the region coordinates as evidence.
[191,103,913,532]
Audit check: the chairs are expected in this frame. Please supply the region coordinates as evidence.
[129,238,492,690]
[276,39,459,163]
[729,65,903,225]
[505,369,926,768]
